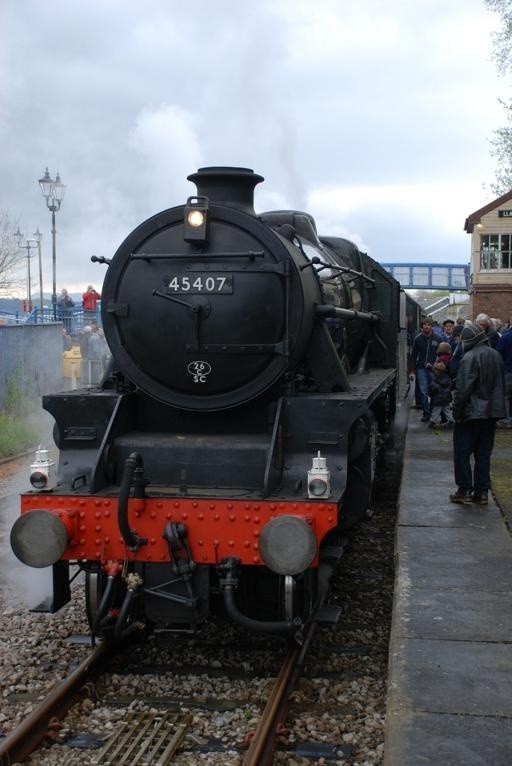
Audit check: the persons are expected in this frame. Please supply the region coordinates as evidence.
[56,289,76,334]
[447,323,507,507]
[60,323,111,387]
[406,311,512,430]
[82,285,102,327]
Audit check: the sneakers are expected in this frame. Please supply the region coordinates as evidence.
[496,419,512,428]
[471,491,489,504]
[421,415,455,429]
[450,490,474,502]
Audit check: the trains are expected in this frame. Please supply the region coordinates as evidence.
[10,165,442,657]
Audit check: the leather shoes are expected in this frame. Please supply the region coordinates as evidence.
[410,404,423,408]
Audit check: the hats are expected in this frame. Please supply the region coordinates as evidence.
[435,362,446,371]
[423,317,434,326]
[460,323,490,352]
[436,342,451,354]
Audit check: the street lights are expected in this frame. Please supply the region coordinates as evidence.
[13,165,66,323]
